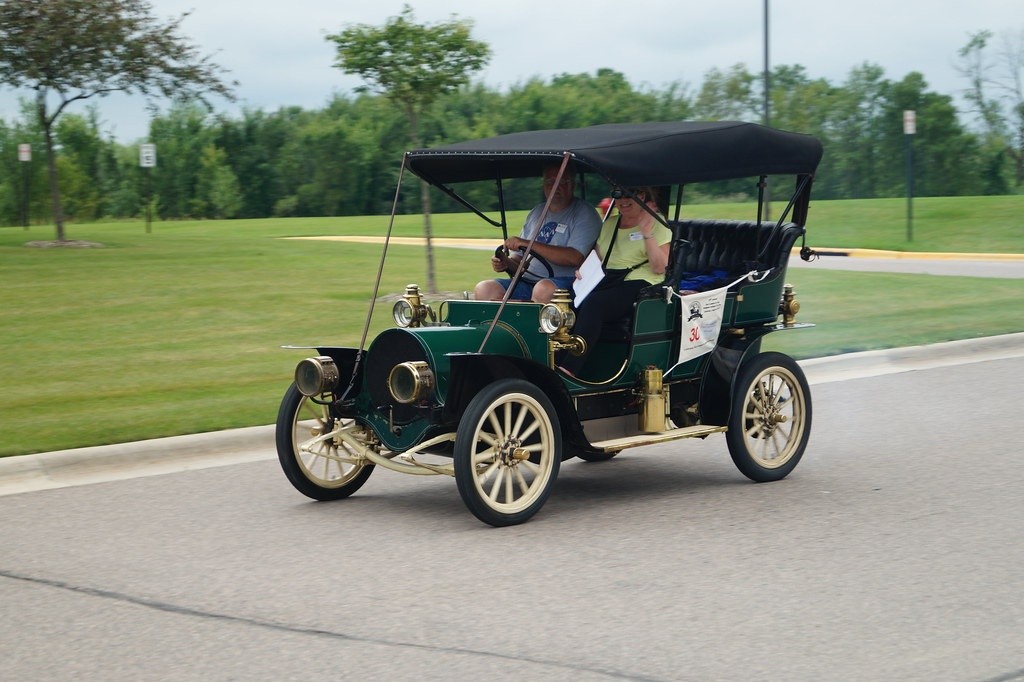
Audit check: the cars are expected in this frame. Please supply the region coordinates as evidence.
[275,119,848,528]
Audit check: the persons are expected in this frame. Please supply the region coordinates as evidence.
[553,183,673,378]
[473,162,603,302]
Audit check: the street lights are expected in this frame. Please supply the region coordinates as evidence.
[16,141,32,233]
[900,106,918,245]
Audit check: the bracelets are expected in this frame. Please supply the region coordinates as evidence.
[644,237,654,239]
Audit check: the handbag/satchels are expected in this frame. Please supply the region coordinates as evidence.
[593,268,632,291]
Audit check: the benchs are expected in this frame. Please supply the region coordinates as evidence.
[667,222,805,292]
[609,273,676,315]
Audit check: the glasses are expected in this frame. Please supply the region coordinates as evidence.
[610,187,635,199]
